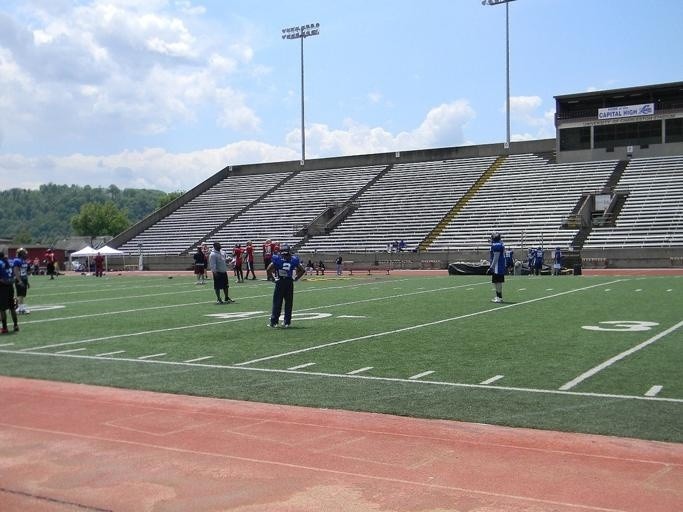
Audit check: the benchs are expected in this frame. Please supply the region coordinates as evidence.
[117,152,682,255]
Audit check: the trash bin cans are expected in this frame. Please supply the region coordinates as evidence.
[574,264,581,275]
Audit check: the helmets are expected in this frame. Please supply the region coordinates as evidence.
[489,233,500,242]
[279,243,289,252]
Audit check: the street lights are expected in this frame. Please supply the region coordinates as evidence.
[280,22,320,160]
[481,0,517,143]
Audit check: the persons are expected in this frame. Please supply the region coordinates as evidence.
[529,247,543,277]
[551,247,561,277]
[386,243,392,254]
[192,239,325,326]
[335,250,343,276]
[504,248,514,275]
[0,246,104,335]
[485,232,504,302]
[392,240,398,251]
[398,240,404,250]
[526,246,534,277]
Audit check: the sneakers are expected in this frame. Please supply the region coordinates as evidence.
[489,296,503,302]
[215,298,235,304]
